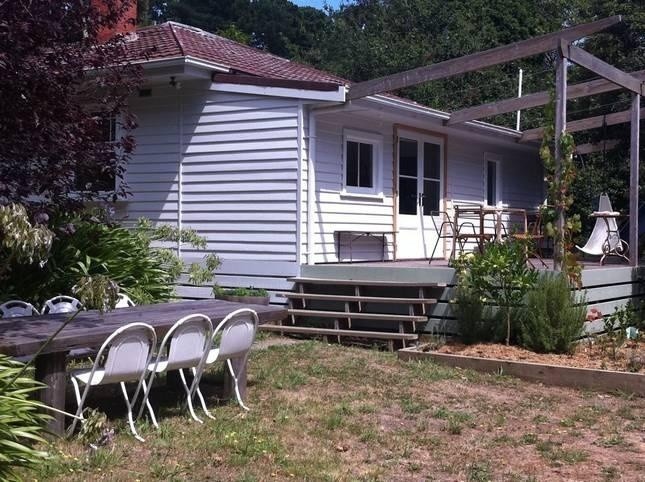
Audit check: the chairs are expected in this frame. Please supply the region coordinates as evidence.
[429,211,548,269]
[0,296,94,375]
[66,308,258,439]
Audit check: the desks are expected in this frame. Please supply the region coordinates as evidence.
[0,298,289,440]
[589,212,630,266]
[453,205,527,265]
[334,229,400,262]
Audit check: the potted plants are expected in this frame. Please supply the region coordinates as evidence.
[213,283,271,305]
